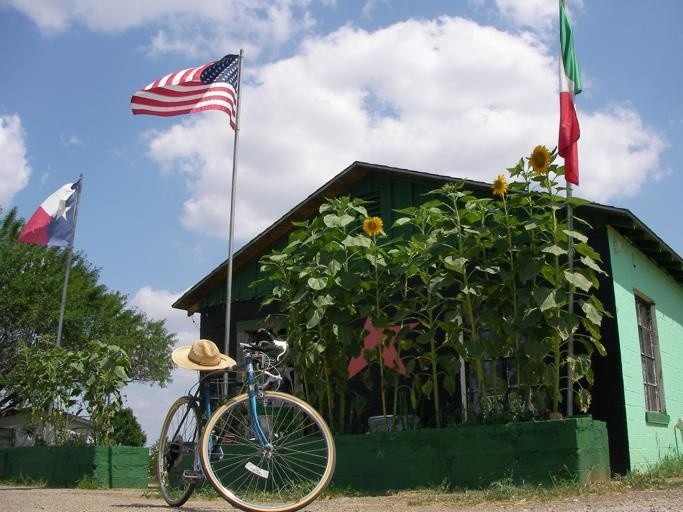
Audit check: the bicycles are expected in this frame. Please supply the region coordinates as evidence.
[158,329,336,512]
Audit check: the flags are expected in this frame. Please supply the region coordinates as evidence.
[130,54,240,130]
[18,176,81,250]
[558,1,583,186]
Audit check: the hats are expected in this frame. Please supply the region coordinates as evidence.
[173,340,237,371]
